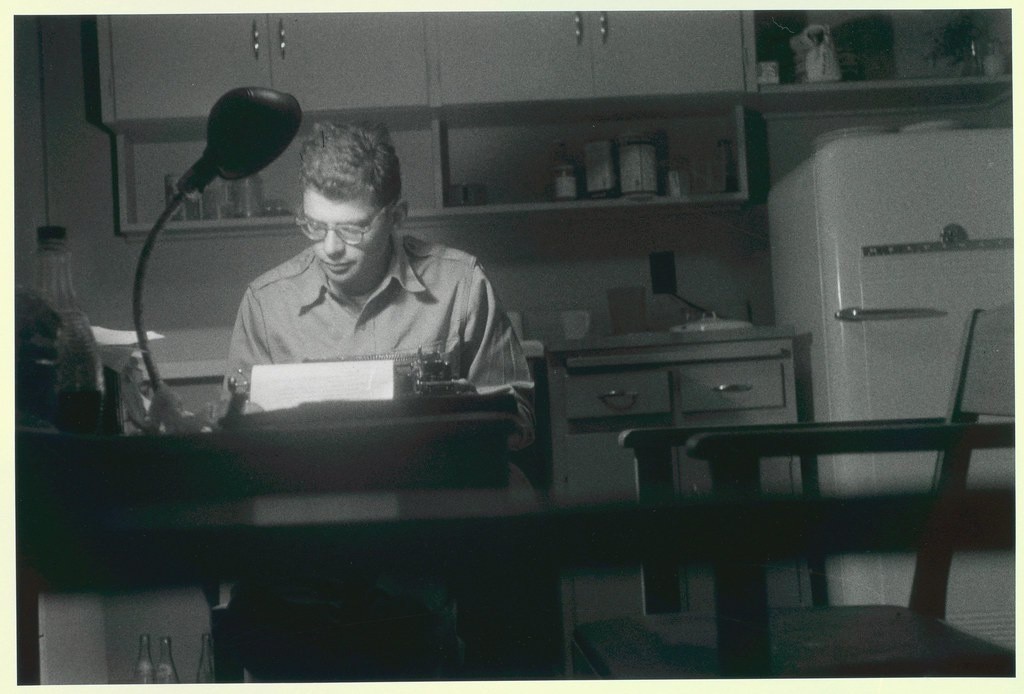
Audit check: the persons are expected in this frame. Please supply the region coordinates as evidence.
[217,120,536,449]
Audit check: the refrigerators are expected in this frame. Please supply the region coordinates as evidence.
[765,122,1014,656]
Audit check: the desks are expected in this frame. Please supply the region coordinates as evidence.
[17,391,566,681]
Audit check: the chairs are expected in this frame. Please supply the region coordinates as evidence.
[566,295,1016,682]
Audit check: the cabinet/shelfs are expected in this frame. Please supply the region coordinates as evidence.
[532,342,818,631]
[86,8,766,238]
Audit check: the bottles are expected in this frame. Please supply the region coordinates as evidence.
[155,638,178,684]
[165,169,268,222]
[197,631,216,684]
[546,125,735,203]
[132,633,155,684]
[34,222,106,430]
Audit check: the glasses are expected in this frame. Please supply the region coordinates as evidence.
[294,196,397,245]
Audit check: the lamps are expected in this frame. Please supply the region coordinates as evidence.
[127,85,304,434]
